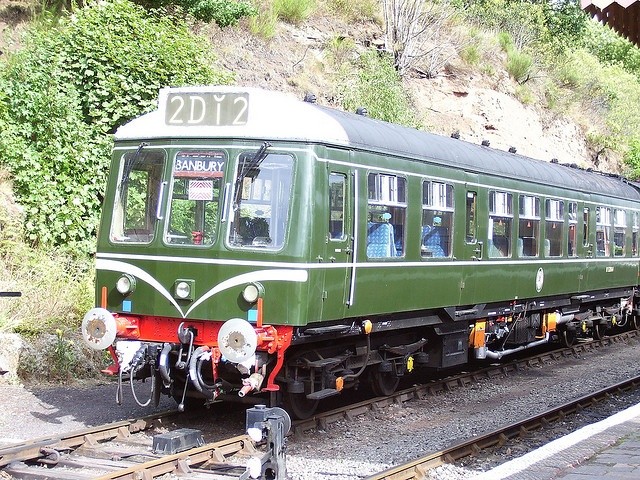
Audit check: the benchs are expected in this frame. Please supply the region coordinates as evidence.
[328,220,396,258]
[522,237,550,255]
[392,224,449,257]
[494,234,523,257]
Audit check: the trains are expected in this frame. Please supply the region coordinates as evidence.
[81,86,640,420]
